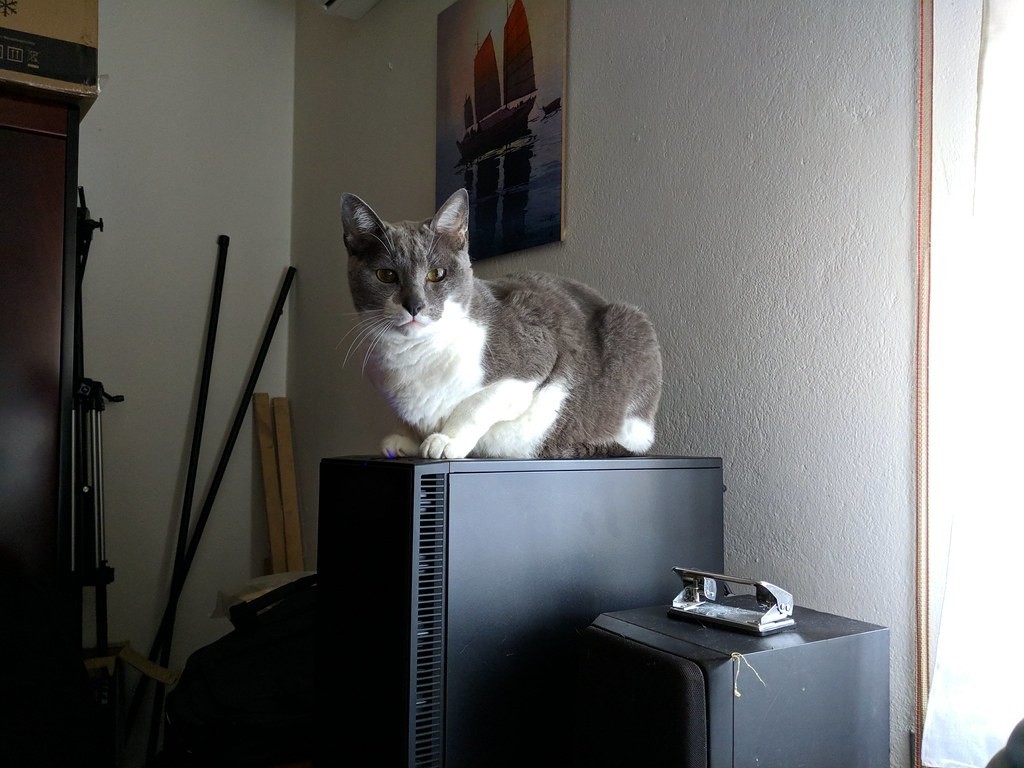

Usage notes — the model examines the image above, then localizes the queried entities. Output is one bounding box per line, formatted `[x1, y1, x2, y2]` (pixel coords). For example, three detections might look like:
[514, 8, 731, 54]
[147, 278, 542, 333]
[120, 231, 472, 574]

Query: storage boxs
[0, 0, 100, 122]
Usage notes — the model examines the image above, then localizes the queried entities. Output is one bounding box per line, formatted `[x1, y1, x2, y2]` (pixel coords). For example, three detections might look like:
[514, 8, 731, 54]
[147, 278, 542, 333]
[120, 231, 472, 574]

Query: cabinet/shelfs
[0, 81, 83, 768]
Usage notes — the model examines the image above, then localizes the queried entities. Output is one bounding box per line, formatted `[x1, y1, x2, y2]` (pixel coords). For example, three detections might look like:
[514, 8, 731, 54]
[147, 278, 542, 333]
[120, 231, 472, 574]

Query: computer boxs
[299, 453, 723, 768]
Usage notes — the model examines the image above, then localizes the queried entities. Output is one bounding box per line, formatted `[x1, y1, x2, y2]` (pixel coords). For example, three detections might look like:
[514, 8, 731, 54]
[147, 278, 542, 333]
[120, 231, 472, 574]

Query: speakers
[585, 600, 891, 768]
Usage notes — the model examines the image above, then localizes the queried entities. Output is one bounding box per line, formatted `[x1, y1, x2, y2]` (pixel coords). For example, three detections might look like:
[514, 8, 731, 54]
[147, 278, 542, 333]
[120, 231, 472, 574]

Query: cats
[335, 187, 663, 459]
